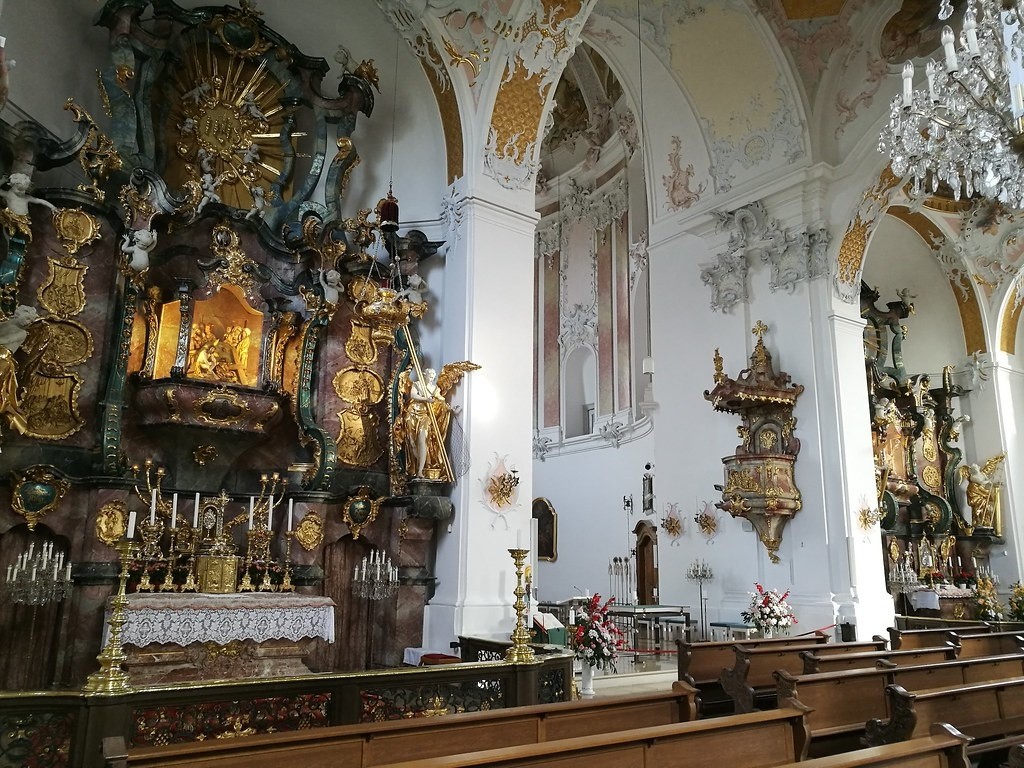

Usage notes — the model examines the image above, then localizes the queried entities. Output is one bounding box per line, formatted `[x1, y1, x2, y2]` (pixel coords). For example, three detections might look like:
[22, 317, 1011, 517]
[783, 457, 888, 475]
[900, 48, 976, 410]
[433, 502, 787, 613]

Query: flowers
[740, 582, 799, 633]
[954, 604, 966, 617]
[1006, 580, 1024, 621]
[925, 572, 945, 583]
[975, 578, 1004, 615]
[955, 572, 976, 584]
[570, 592, 625, 673]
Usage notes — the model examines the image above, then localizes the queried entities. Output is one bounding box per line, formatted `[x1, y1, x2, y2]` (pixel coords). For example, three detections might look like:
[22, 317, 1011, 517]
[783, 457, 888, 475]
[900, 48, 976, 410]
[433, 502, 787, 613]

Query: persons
[896, 288, 918, 315]
[875, 398, 890, 431]
[924, 409, 937, 431]
[178, 79, 270, 220]
[317, 266, 344, 322]
[395, 273, 429, 305]
[405, 369, 445, 478]
[966, 463, 995, 528]
[0, 304, 37, 435]
[186, 322, 251, 382]
[121, 230, 158, 292]
[0, 173, 59, 244]
[334, 45, 382, 95]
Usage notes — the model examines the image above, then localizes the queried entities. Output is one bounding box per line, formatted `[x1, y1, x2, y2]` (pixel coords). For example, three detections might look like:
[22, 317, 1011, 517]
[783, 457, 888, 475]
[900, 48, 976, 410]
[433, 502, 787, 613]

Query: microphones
[574, 586, 583, 597]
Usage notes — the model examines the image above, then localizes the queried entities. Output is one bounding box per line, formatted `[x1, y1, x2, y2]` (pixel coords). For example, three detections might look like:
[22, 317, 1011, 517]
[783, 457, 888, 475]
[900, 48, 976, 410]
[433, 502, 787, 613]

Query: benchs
[103, 618, 1024, 768]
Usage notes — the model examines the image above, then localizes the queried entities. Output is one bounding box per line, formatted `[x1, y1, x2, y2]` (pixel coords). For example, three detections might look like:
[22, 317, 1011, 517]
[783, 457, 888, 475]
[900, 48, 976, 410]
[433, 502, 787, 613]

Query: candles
[127, 511, 137, 538]
[354, 548, 398, 583]
[948, 557, 953, 568]
[171, 492, 177, 528]
[150, 487, 157, 525]
[971, 557, 1000, 583]
[888, 563, 918, 584]
[928, 555, 933, 567]
[192, 493, 200, 527]
[287, 498, 293, 533]
[6, 540, 73, 582]
[248, 495, 254, 529]
[516, 525, 523, 547]
[958, 556, 962, 567]
[688, 557, 713, 578]
[268, 495, 274, 530]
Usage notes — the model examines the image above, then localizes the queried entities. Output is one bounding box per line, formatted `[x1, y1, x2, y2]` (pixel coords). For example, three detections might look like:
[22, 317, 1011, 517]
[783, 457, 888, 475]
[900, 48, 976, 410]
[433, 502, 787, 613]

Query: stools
[549, 604, 565, 622]
[537, 603, 548, 615]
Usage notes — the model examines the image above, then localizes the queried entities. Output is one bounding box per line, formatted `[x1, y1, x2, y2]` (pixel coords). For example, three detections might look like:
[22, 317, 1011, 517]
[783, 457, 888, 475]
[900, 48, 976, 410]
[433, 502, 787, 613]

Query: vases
[578, 659, 595, 699]
[935, 583, 940, 588]
[959, 582, 966, 588]
[928, 584, 930, 587]
[969, 584, 977, 588]
[981, 610, 993, 620]
[764, 624, 773, 638]
[954, 616, 962, 620]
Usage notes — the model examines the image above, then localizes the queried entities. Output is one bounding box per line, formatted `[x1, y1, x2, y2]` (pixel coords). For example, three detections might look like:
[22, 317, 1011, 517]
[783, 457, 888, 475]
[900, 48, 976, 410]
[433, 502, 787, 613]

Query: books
[533, 613, 565, 632]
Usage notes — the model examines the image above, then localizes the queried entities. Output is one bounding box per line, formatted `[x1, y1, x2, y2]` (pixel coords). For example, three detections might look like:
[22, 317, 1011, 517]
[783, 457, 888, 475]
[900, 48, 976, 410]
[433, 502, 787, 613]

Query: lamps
[637, 1, 655, 375]
[878, 0, 1023, 210]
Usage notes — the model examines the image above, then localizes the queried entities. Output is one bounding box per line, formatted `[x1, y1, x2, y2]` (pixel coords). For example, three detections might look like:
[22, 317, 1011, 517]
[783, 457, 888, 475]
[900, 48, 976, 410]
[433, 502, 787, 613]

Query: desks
[102, 594, 335, 680]
[602, 604, 691, 663]
